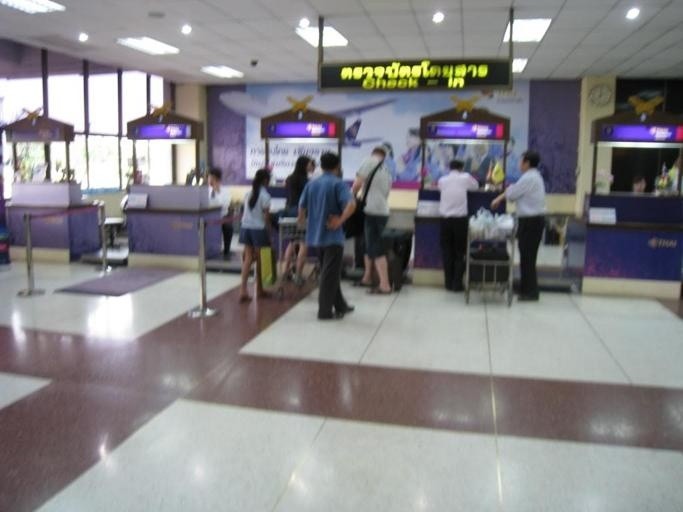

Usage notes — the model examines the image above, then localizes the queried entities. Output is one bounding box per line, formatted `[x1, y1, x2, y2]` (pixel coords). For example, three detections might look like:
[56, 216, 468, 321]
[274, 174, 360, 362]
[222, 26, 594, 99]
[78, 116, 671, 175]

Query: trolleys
[460, 207, 517, 308]
[275, 213, 325, 302]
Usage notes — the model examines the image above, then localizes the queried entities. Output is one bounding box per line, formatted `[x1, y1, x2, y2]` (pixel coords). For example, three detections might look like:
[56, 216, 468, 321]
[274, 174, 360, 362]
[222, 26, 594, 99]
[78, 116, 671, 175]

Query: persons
[629, 174, 646, 194]
[275, 145, 393, 321]
[238, 170, 274, 303]
[205, 166, 235, 259]
[490, 150, 547, 302]
[436, 157, 480, 293]
[383, 126, 523, 190]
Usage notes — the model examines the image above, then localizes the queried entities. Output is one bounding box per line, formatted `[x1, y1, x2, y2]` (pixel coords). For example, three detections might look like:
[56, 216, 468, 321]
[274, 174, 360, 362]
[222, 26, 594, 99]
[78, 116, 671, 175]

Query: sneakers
[281, 271, 304, 285]
[319, 307, 353, 318]
[239, 289, 271, 301]
[353, 280, 391, 294]
[514, 285, 539, 300]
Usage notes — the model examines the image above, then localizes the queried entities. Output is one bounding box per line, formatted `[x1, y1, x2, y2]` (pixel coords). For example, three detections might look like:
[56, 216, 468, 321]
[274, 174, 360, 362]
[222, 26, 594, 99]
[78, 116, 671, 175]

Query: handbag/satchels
[272, 211, 287, 228]
[342, 197, 366, 238]
[259, 245, 277, 287]
